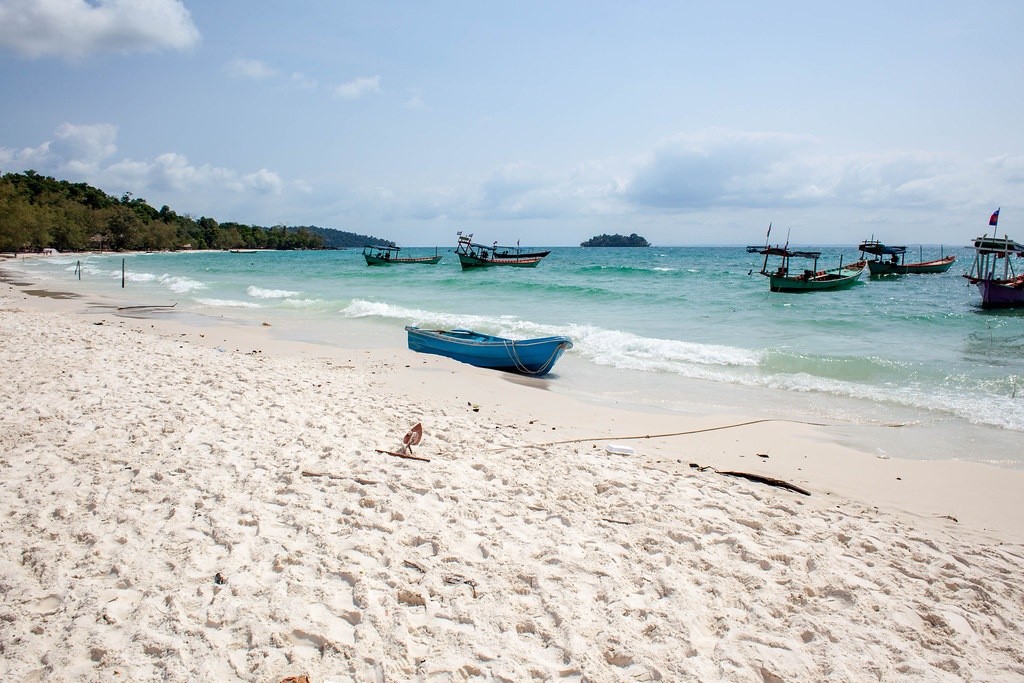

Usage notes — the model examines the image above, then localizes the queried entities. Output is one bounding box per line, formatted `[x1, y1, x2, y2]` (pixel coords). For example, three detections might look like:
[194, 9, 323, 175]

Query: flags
[989, 209, 999, 226]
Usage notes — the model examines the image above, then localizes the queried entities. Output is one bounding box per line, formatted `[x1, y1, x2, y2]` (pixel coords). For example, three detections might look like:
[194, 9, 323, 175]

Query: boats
[362, 243, 443, 267]
[404, 325, 574, 378]
[963, 234, 1024, 311]
[760, 221, 867, 293]
[455, 230, 552, 269]
[866, 239, 956, 276]
[858, 233, 909, 255]
[230, 249, 258, 253]
[746, 245, 821, 259]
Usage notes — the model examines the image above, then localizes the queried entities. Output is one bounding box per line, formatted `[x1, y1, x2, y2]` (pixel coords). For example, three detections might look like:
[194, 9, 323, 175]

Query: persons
[804, 270, 808, 277]
[15, 253, 16, 258]
[891, 254, 895, 262]
[43, 250, 52, 255]
[483, 251, 487, 258]
[385, 252, 390, 258]
